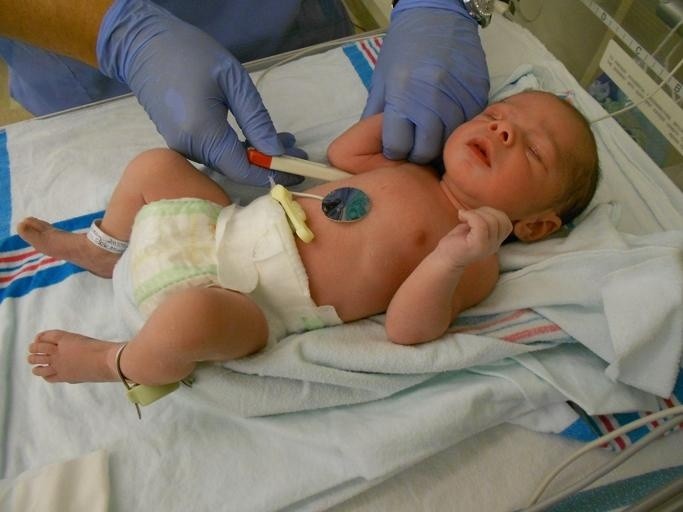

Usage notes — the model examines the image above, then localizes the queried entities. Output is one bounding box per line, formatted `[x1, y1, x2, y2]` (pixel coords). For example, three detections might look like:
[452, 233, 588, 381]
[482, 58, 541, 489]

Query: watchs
[461, 0, 492, 27]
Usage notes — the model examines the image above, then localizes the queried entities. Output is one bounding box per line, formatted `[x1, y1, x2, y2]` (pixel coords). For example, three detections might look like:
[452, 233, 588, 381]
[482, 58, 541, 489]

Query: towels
[112, 64, 683, 416]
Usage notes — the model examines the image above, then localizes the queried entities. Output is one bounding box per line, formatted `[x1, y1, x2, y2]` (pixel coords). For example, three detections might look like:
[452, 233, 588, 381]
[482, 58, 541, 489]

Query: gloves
[360, 0, 491, 165]
[96, 0, 309, 189]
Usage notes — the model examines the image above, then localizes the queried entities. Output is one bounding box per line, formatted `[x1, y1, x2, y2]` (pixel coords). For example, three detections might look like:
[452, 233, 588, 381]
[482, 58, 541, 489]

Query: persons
[14, 89, 602, 388]
[1, 0, 493, 187]
